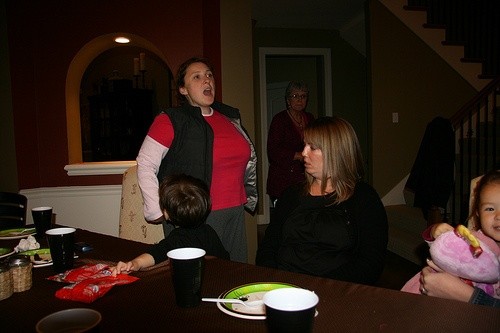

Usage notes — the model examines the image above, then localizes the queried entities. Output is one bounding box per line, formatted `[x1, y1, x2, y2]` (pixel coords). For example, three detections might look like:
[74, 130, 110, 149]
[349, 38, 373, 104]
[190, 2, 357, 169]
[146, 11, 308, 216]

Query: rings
[421, 288, 424, 293]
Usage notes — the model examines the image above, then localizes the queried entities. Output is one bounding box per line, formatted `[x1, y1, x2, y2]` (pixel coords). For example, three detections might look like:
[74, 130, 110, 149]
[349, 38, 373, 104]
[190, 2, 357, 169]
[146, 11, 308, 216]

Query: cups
[32, 206, 53, 231]
[35, 308, 102, 333]
[264, 287, 319, 333]
[167, 247, 206, 309]
[45, 228, 76, 272]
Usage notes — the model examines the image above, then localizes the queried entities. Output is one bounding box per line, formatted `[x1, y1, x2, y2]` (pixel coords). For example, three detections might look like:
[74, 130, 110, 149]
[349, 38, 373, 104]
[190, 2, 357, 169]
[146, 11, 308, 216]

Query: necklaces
[288, 109, 304, 127]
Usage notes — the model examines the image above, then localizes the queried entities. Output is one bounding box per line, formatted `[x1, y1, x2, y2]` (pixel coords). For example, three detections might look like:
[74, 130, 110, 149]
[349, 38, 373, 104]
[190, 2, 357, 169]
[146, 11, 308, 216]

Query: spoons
[202, 298, 262, 306]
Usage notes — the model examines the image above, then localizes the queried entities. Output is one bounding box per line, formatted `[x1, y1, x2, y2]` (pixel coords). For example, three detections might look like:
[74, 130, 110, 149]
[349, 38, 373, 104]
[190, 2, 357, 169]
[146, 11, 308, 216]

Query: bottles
[0, 259, 14, 300]
[8, 254, 33, 293]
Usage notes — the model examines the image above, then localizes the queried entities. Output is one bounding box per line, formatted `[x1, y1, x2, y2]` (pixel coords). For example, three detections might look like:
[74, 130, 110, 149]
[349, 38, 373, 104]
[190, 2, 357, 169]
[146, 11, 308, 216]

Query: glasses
[288, 93, 308, 99]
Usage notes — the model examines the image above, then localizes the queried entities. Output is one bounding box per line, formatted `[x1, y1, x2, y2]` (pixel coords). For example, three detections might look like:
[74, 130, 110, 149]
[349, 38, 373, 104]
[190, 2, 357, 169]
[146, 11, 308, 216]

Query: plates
[0, 247, 16, 258]
[0, 228, 36, 239]
[18, 248, 53, 267]
[216, 282, 303, 320]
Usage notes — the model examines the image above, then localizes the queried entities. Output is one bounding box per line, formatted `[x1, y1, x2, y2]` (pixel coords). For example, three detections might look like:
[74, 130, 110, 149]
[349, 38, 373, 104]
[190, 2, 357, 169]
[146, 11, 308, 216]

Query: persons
[264, 81, 317, 216]
[400, 170, 500, 308]
[136, 59, 259, 263]
[254, 116, 388, 287]
[418, 259, 500, 307]
[112, 184, 230, 279]
